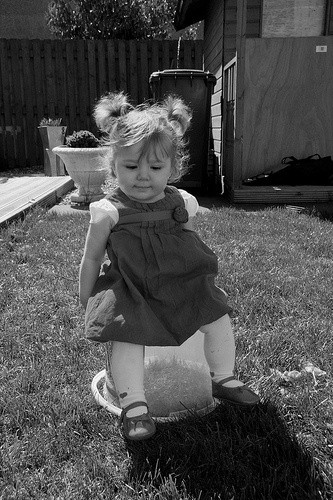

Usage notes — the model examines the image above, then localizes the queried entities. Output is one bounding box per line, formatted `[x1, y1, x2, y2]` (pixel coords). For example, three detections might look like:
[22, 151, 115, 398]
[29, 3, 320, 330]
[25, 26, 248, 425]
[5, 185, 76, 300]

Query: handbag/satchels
[282, 154, 333, 187]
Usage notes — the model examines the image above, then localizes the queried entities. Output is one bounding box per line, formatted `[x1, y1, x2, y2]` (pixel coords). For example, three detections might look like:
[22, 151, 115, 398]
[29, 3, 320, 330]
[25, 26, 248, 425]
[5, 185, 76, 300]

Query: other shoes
[121, 401, 155, 439]
[211, 376, 260, 405]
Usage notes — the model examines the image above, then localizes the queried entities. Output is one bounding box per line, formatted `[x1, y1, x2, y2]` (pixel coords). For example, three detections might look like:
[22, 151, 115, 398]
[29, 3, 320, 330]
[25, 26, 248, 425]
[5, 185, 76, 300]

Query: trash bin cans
[149, 68, 221, 198]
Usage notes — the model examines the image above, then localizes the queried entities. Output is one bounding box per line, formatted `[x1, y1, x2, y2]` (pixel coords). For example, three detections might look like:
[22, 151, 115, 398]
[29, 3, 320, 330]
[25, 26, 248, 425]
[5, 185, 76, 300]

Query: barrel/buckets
[91, 328, 229, 424]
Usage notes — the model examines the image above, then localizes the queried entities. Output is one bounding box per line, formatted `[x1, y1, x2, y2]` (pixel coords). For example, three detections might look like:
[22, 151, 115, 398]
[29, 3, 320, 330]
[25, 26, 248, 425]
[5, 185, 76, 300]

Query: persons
[79, 91, 261, 440]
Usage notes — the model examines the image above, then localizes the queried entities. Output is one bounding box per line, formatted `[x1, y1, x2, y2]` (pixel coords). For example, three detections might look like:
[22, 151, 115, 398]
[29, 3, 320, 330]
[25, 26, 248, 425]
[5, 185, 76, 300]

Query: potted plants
[51, 131, 112, 203]
[37, 118, 67, 177]
